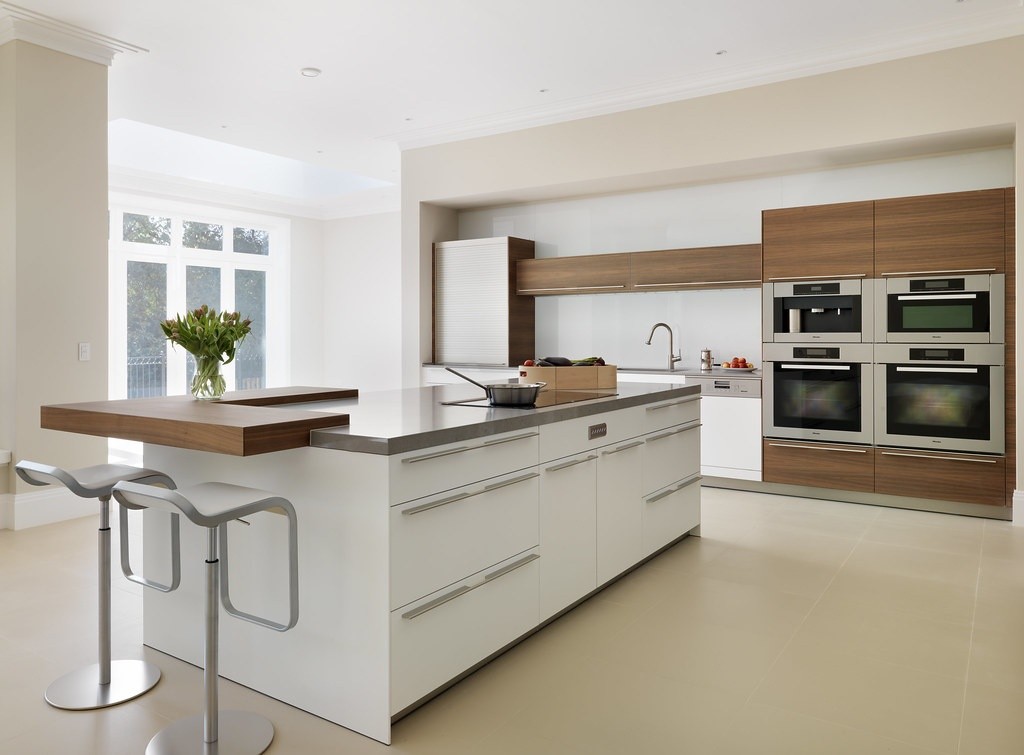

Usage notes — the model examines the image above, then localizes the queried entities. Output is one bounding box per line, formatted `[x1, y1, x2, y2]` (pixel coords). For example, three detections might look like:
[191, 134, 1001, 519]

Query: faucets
[646, 322, 682, 371]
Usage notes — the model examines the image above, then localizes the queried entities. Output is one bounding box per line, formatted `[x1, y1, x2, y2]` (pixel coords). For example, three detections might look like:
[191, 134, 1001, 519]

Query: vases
[190, 355, 225, 401]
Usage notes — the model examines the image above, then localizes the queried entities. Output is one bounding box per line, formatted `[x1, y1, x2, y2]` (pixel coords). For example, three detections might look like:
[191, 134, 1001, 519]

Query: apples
[722, 357, 752, 368]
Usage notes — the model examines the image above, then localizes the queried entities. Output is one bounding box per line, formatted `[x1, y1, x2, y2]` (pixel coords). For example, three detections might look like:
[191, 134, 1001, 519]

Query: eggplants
[533, 356, 592, 367]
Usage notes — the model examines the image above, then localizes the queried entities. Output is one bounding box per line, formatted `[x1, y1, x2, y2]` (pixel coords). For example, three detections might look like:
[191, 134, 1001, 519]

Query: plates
[722, 367, 758, 372]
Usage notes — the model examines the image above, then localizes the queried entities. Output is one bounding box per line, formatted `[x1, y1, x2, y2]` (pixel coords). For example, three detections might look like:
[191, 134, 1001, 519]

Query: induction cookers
[441, 389, 619, 410]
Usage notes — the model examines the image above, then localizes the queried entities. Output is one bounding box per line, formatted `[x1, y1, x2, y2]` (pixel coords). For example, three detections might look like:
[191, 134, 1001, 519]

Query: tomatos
[521, 360, 534, 377]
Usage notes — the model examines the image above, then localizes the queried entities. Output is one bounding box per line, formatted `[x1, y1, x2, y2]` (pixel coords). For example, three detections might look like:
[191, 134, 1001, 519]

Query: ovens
[762, 267, 1007, 454]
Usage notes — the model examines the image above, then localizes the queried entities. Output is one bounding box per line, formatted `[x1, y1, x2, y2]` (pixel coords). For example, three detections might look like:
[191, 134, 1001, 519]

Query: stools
[15, 458, 182, 712]
[112, 476, 298, 755]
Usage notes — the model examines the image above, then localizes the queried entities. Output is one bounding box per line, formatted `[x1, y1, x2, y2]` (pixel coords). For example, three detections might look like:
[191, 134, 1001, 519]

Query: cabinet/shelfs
[760, 187, 1004, 283]
[516, 243, 763, 293]
[535, 404, 646, 465]
[642, 421, 703, 562]
[310, 428, 540, 716]
[539, 434, 642, 629]
[431, 235, 535, 367]
[762, 437, 1006, 510]
[644, 389, 701, 435]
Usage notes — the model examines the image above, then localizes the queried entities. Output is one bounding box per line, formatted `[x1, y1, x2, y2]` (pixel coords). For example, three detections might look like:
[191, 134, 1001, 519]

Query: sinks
[617, 367, 667, 371]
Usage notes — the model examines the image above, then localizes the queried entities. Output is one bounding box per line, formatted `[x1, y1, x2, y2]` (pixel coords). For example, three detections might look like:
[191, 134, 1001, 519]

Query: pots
[446, 367, 547, 405]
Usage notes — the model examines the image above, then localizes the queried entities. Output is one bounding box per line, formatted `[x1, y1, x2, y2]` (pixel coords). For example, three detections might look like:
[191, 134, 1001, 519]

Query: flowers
[159, 304, 259, 397]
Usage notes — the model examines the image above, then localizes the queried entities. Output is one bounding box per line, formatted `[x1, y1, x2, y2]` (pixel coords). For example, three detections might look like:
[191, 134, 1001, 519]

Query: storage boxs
[518, 365, 617, 390]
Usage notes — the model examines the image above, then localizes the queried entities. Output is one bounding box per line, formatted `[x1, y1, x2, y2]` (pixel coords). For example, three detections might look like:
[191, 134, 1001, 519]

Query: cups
[700, 348, 714, 370]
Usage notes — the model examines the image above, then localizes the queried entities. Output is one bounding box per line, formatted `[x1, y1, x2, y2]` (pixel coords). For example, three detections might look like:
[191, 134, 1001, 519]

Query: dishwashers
[685, 370, 763, 481]
